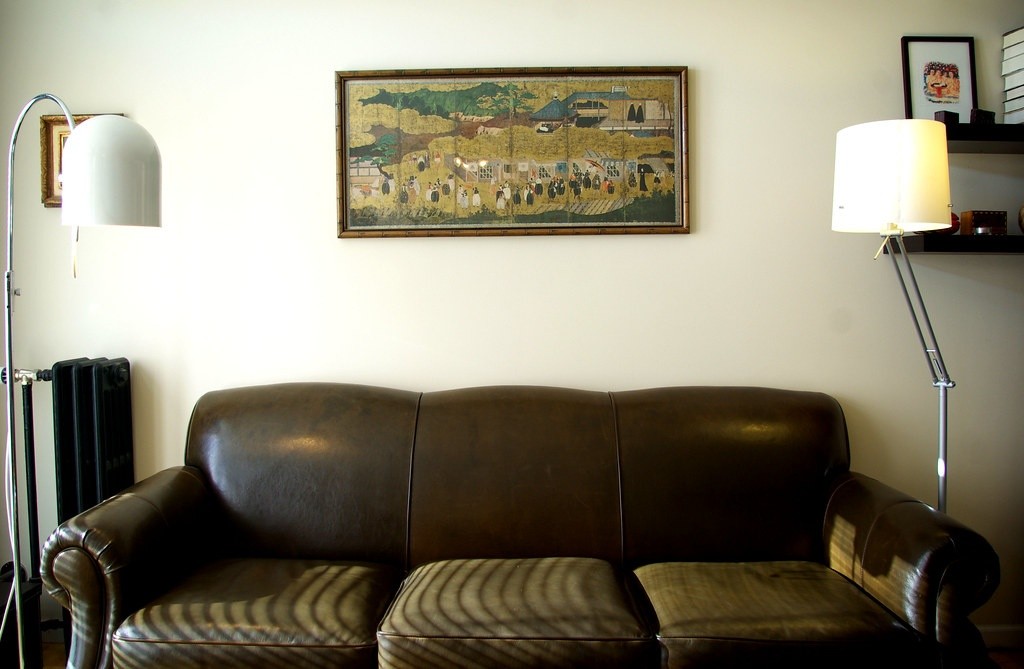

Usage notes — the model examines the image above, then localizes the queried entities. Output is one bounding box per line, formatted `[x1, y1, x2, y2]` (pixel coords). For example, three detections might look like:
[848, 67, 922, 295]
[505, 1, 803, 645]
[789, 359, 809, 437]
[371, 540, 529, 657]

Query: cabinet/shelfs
[883, 123, 1024, 255]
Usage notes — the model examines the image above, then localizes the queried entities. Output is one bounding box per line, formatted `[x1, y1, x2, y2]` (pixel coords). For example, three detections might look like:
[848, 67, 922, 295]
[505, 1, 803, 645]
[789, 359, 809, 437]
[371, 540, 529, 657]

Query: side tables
[0, 582, 42, 669]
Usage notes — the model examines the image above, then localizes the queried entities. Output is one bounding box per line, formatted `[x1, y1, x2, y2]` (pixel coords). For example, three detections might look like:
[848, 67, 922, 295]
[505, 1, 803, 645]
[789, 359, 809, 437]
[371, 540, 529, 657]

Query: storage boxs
[960, 210, 1008, 236]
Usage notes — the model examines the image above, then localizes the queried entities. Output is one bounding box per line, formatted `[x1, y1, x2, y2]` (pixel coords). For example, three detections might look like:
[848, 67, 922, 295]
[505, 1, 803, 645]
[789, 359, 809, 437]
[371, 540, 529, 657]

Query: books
[1001, 27, 1024, 124]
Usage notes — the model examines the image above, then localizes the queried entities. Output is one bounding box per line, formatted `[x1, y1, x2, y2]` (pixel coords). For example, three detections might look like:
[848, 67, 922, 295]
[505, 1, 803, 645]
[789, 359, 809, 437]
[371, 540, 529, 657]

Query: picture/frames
[39, 112, 124, 208]
[334, 65, 691, 238]
[901, 35, 979, 123]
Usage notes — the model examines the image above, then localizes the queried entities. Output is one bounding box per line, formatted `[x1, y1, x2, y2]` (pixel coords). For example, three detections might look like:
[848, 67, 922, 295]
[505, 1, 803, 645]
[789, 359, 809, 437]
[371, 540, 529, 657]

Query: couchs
[39, 382, 1001, 669]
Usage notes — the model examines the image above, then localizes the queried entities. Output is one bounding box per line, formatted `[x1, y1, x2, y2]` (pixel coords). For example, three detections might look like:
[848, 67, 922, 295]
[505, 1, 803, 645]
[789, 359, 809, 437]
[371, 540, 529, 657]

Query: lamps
[4, 93, 163, 669]
[831, 118, 957, 511]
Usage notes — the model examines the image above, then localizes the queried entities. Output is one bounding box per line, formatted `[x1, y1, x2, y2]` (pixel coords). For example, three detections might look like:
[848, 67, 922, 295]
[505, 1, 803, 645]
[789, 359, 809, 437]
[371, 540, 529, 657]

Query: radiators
[0, 357, 135, 669]
